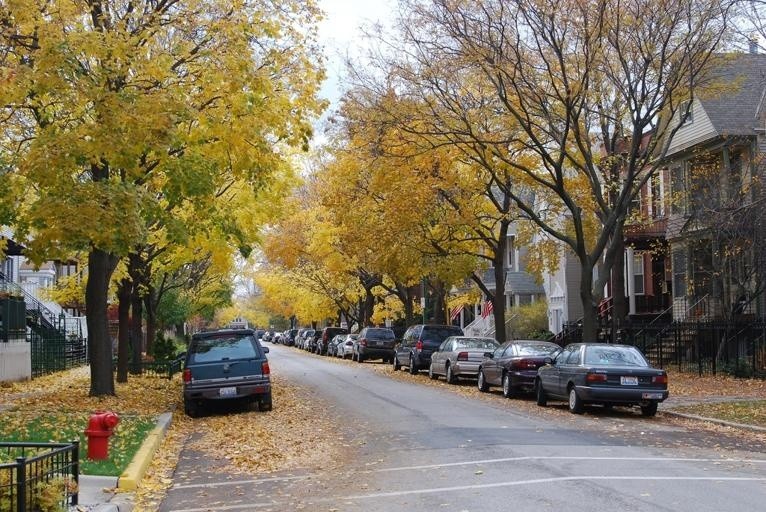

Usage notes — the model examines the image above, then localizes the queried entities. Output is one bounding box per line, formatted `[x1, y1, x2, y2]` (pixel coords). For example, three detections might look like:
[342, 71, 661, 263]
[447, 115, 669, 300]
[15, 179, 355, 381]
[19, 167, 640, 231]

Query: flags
[450, 303, 464, 320]
[481, 298, 494, 319]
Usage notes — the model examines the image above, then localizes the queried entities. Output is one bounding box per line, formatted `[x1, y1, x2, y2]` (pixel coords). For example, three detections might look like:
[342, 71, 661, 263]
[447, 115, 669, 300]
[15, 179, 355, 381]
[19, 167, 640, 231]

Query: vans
[182, 327, 272, 417]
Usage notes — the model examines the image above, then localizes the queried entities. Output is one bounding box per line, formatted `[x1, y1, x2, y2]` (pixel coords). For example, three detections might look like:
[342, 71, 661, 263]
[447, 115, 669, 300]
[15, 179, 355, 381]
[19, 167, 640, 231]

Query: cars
[352, 326, 397, 365]
[477, 339, 563, 399]
[255, 327, 359, 358]
[429, 335, 501, 385]
[392, 324, 465, 375]
[535, 341, 669, 416]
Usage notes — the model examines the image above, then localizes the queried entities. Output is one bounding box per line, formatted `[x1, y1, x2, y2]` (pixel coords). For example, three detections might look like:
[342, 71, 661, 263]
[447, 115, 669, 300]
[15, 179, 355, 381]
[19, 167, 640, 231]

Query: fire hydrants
[84, 410, 120, 460]
